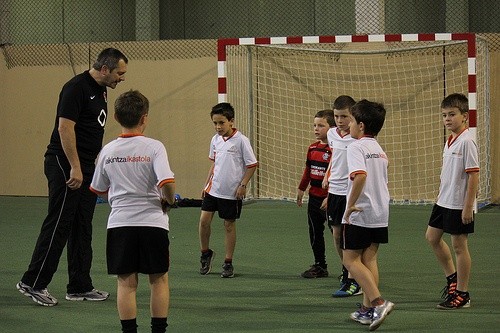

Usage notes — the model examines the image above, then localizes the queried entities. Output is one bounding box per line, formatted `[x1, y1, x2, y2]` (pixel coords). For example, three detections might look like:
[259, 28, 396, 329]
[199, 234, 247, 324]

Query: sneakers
[16, 280, 58, 307]
[65, 288, 110, 300]
[301, 263, 328, 278]
[220, 261, 235, 278]
[436, 289, 473, 309]
[199, 249, 216, 275]
[332, 281, 363, 297]
[365, 299, 394, 330]
[440, 280, 457, 303]
[349, 303, 372, 324]
[338, 267, 348, 287]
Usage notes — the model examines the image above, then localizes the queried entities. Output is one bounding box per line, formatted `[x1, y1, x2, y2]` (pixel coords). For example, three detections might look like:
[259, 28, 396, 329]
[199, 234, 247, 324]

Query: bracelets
[240, 183, 246, 189]
[166, 202, 174, 208]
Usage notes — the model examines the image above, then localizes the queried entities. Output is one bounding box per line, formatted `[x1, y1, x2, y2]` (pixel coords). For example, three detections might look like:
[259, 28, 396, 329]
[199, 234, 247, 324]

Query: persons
[16, 48, 129, 306]
[425, 93, 480, 309]
[340, 99, 395, 332]
[322, 95, 363, 298]
[198, 103, 258, 278]
[89, 89, 175, 333]
[297, 110, 337, 278]
